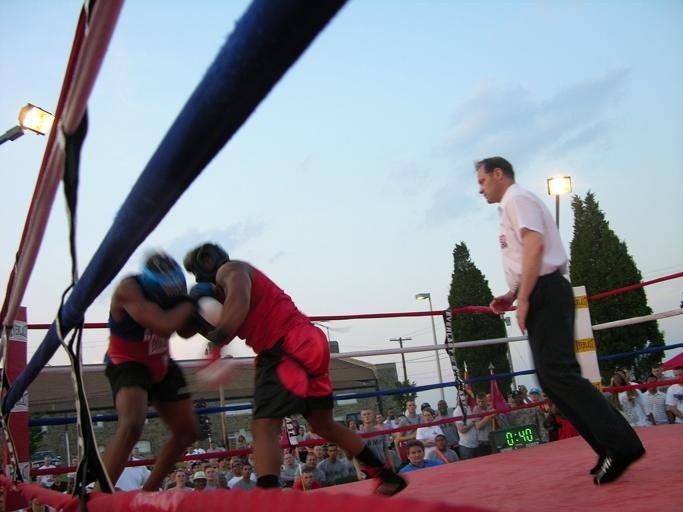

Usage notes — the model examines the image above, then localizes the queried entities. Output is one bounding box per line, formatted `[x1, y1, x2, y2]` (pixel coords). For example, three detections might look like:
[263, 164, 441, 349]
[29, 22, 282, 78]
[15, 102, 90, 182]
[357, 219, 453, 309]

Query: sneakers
[592, 440, 649, 486]
[587, 452, 605, 474]
[371, 470, 410, 499]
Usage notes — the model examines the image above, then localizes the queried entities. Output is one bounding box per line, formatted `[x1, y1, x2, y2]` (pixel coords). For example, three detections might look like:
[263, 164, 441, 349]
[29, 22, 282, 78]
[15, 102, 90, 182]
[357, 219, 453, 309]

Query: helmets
[141, 253, 186, 306]
[183, 241, 229, 280]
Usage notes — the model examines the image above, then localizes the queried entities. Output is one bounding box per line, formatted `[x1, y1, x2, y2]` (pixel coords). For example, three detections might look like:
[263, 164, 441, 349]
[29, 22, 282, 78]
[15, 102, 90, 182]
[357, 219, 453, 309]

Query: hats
[420, 402, 431, 410]
[192, 471, 207, 481]
[529, 388, 540, 395]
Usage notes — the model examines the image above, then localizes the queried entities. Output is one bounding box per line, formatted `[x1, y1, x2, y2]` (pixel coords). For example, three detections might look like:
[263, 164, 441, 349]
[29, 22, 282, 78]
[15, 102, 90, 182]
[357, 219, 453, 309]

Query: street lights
[413, 291, 445, 403]
[547, 173, 571, 229]
[388, 337, 412, 396]
[0, 101, 54, 145]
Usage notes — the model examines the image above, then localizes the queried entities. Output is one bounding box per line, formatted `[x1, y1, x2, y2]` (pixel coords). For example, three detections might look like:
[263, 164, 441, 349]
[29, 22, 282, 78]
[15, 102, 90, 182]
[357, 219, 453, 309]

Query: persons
[93, 253, 218, 492]
[37, 387, 564, 497]
[476, 157, 647, 485]
[184, 242, 407, 497]
[609, 356, 683, 426]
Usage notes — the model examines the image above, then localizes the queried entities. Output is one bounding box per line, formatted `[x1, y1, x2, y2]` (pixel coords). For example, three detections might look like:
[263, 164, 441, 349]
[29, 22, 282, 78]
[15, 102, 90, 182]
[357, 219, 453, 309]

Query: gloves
[188, 282, 226, 305]
[193, 296, 223, 336]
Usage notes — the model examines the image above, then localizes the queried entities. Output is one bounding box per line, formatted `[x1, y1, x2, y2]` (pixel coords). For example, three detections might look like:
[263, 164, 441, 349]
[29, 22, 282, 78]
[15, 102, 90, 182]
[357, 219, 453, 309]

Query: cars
[29, 448, 63, 469]
